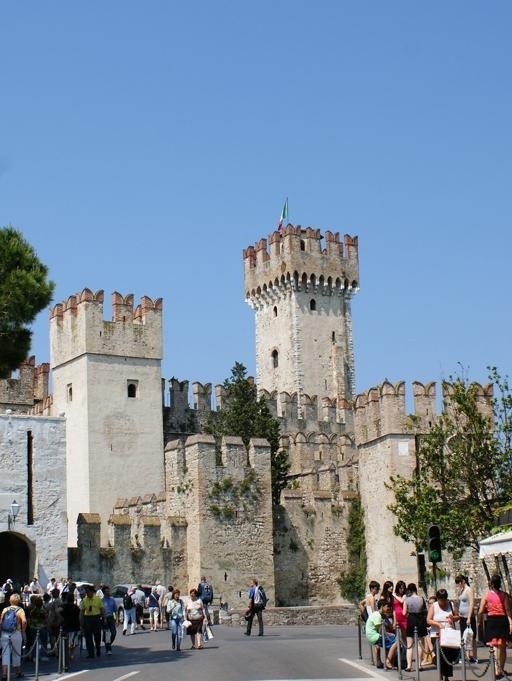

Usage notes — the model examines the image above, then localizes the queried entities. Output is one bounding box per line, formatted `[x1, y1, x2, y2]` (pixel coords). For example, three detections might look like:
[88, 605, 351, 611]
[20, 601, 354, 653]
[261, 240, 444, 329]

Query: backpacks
[0, 605, 22, 634]
[254, 585, 269, 610]
[5, 584, 11, 591]
[123, 592, 135, 610]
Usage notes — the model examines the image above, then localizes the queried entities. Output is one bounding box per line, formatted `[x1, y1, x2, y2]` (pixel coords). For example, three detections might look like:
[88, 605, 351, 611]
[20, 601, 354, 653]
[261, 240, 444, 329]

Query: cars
[47, 581, 104, 606]
[111, 582, 171, 625]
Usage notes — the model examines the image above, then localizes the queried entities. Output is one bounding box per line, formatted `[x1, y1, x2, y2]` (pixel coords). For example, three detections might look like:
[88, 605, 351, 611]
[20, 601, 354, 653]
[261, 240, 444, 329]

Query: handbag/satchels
[202, 620, 215, 644]
[420, 596, 432, 628]
[439, 617, 462, 649]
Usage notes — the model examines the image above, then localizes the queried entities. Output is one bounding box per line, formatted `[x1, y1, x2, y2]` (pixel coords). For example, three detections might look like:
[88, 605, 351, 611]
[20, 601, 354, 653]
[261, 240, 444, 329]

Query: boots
[1, 665, 8, 680]
[14, 665, 24, 678]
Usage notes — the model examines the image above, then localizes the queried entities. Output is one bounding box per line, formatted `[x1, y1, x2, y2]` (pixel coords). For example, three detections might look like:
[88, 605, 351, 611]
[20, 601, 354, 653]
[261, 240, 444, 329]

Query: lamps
[8, 499, 21, 524]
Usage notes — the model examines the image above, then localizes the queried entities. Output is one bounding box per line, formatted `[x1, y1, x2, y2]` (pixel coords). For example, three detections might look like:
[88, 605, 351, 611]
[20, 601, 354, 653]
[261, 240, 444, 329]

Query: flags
[278, 200, 287, 230]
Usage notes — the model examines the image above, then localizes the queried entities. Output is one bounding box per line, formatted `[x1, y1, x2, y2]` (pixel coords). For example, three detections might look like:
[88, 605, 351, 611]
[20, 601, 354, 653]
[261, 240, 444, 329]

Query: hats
[6, 578, 13, 583]
[245, 611, 253, 622]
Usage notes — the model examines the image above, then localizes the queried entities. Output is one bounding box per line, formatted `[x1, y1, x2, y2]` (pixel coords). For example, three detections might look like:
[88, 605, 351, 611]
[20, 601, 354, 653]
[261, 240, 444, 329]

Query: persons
[244, 579, 263, 636]
[455, 575, 476, 663]
[323, 249, 327, 255]
[477, 575, 512, 679]
[359, 574, 461, 681]
[0, 576, 214, 681]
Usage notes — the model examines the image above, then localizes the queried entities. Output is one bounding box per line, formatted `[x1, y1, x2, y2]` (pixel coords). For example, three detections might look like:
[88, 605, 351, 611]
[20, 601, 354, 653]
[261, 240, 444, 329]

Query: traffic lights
[427, 524, 442, 562]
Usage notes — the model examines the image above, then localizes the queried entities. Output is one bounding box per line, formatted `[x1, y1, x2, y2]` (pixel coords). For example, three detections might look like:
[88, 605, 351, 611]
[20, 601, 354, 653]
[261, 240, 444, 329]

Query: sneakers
[191, 644, 204, 650]
[172, 643, 181, 652]
[244, 632, 251, 636]
[122, 624, 171, 636]
[496, 670, 509, 680]
[32, 651, 113, 664]
[258, 634, 264, 637]
[377, 663, 423, 672]
[465, 656, 481, 664]
